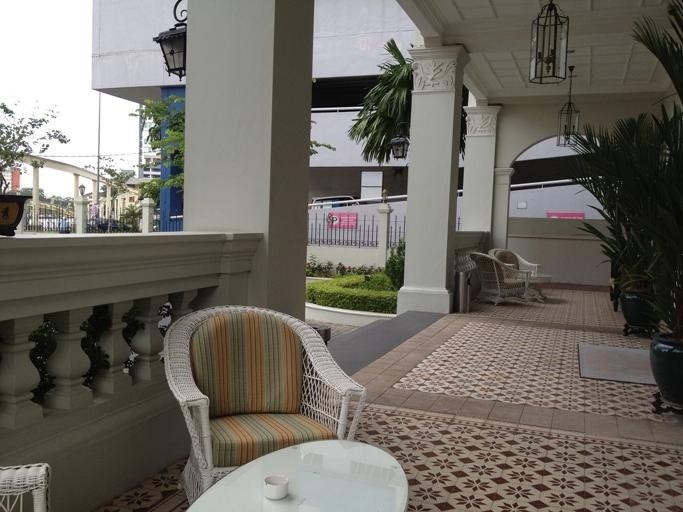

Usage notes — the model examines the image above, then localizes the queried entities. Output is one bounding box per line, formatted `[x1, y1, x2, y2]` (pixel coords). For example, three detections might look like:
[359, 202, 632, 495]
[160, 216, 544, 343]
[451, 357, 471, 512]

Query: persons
[58, 217, 72, 233]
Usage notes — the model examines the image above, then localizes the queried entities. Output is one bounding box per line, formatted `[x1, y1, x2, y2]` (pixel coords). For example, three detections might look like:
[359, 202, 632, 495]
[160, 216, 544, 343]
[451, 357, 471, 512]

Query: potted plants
[561, 101, 682, 405]
[0, 101, 69, 235]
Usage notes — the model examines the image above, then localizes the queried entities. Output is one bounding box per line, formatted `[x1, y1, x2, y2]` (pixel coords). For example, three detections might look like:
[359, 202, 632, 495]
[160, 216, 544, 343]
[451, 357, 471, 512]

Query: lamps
[154, 0, 187, 82]
[388, 122, 410, 158]
[531, 1, 581, 147]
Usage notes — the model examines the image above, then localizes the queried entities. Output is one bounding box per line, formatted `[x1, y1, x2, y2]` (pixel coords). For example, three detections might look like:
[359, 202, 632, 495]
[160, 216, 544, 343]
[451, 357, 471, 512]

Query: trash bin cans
[300, 322, 331, 416]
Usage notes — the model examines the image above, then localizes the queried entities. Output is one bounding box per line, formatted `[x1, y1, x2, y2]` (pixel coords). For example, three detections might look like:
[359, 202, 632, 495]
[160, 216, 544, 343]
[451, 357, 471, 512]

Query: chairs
[163, 304, 368, 508]
[470, 248, 545, 307]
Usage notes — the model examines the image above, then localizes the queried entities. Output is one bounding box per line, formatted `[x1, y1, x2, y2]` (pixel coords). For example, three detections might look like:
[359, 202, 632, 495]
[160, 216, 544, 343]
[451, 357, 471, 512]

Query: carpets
[578, 342, 657, 386]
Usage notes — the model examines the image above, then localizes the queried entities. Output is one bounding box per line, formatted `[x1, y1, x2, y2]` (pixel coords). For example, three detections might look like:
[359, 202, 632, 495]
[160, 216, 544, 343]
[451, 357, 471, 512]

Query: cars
[152, 219, 159, 232]
[58, 217, 96, 233]
[99, 218, 130, 232]
